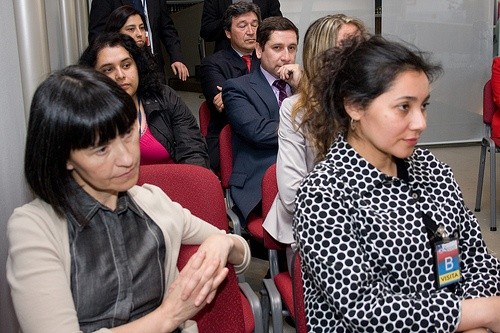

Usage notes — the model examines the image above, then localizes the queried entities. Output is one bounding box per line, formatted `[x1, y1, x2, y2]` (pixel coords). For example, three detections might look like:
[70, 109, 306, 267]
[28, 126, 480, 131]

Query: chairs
[475, 79, 500, 230]
[199, 101, 305, 333]
[136, 164, 263, 333]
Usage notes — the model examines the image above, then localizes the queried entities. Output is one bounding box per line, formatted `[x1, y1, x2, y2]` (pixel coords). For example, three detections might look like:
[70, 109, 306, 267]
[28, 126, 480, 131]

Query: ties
[242, 55, 252, 72]
[272, 80, 288, 106]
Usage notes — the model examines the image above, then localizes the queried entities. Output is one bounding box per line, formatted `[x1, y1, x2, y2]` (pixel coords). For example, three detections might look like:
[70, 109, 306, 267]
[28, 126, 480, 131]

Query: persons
[222, 16, 304, 224]
[77, 34, 208, 169]
[294, 34, 500, 333]
[88, 0, 189, 84]
[262, 16, 365, 246]
[491, 57, 500, 148]
[6, 65, 252, 332]
[200, 0, 283, 134]
